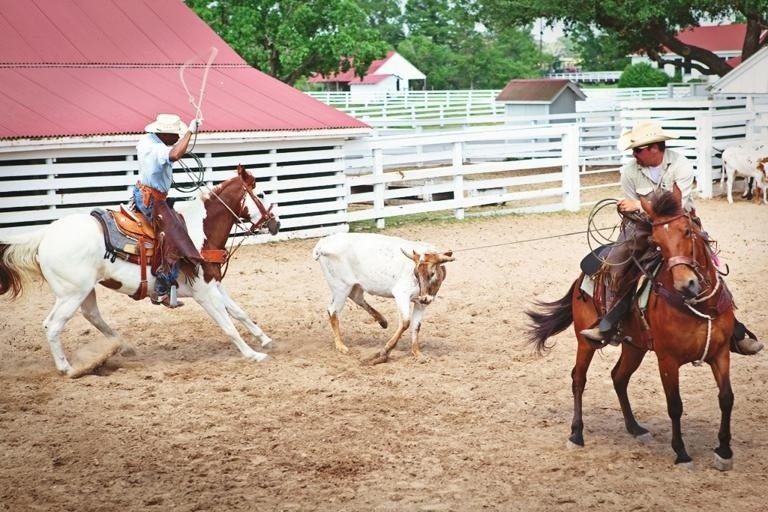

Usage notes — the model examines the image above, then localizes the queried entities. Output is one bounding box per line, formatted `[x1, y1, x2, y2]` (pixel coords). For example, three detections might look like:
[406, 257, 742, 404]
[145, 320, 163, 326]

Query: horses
[523, 180, 740, 472]
[0, 162, 283, 377]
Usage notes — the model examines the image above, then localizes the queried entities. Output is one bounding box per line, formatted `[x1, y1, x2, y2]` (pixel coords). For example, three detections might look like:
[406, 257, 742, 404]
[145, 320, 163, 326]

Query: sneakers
[580, 326, 623, 347]
[730, 337, 764, 354]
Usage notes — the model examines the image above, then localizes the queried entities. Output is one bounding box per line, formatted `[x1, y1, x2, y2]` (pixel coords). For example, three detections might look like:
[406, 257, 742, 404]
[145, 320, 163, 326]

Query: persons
[131, 112, 207, 309]
[579, 121, 764, 357]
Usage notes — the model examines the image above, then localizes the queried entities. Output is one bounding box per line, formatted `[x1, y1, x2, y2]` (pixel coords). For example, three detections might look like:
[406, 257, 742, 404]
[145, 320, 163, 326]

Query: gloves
[188, 119, 204, 134]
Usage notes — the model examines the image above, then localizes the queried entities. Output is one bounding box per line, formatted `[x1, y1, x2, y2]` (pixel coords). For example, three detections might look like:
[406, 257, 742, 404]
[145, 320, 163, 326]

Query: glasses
[633, 144, 654, 153]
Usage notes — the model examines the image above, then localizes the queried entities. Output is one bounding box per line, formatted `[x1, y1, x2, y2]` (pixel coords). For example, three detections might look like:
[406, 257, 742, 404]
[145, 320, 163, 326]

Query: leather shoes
[150, 291, 185, 308]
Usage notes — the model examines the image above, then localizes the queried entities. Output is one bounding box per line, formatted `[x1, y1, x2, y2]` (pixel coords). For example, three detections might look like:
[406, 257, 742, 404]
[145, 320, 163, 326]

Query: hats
[617, 120, 681, 152]
[143, 113, 189, 136]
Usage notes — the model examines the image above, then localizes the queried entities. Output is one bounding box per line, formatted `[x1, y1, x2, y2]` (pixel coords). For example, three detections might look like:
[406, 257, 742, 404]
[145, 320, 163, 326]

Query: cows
[310, 229, 457, 364]
[718, 146, 768, 206]
